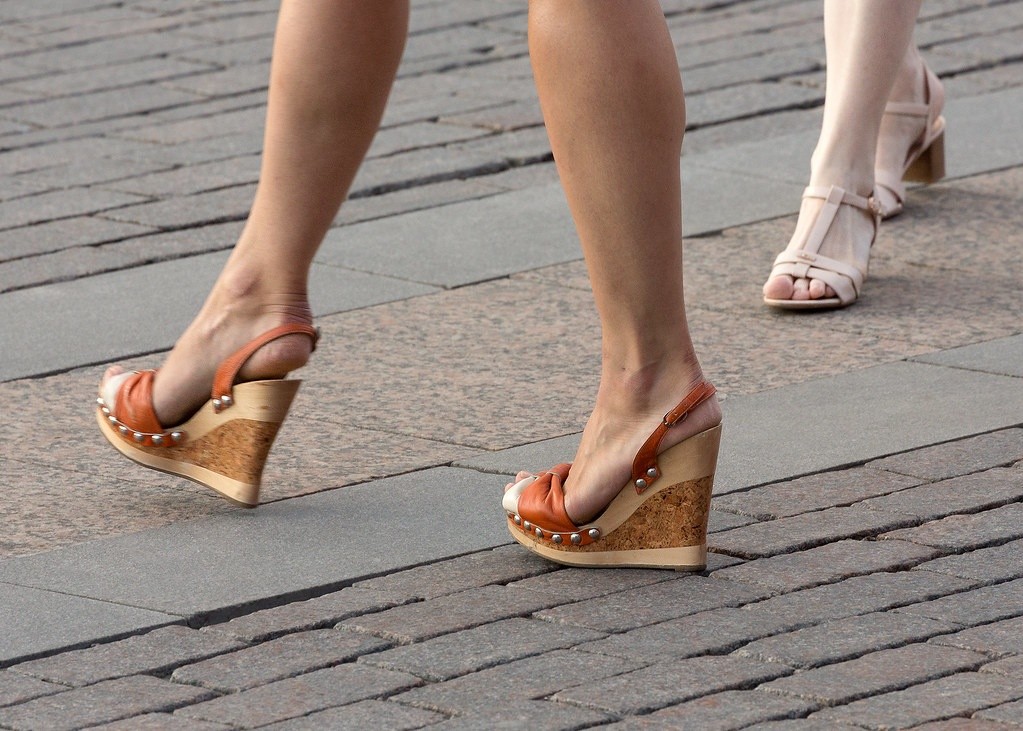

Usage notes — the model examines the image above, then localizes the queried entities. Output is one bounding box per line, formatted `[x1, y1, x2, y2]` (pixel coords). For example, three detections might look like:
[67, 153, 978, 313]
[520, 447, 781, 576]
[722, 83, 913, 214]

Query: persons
[95, 0, 722, 572]
[763, 0, 945, 307]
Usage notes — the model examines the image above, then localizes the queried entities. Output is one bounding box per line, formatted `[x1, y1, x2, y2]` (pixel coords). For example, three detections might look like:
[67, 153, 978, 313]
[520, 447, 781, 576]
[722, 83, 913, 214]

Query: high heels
[502, 381, 723, 577]
[96, 324, 322, 509]
[762, 185, 888, 310]
[872, 57, 947, 220]
